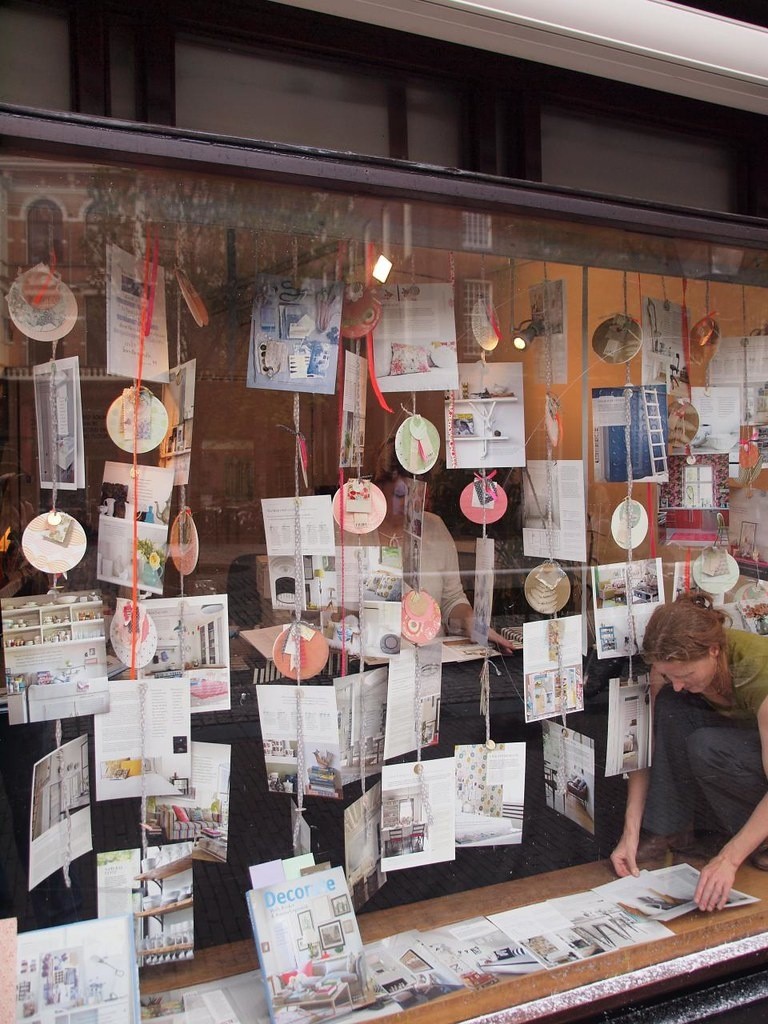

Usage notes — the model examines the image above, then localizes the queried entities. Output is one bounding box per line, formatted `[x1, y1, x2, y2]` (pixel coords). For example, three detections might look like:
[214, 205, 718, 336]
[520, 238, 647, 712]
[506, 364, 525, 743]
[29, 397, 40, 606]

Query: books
[244, 865, 376, 1024]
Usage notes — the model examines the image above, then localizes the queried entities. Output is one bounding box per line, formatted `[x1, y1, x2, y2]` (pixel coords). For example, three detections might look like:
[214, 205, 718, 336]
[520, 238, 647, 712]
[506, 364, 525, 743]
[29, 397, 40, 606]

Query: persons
[325, 437, 515, 648]
[606, 591, 767, 912]
[11, 499, 130, 750]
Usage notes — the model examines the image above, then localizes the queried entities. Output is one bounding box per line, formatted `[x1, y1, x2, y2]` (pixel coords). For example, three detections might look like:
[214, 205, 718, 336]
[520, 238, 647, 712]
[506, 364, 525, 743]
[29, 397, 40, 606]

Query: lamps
[513, 320, 545, 350]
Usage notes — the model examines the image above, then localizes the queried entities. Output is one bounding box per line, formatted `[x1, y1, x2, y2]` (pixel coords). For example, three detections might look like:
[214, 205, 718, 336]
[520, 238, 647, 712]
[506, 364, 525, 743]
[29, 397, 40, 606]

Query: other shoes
[749, 842, 768, 871]
[666, 827, 728, 857]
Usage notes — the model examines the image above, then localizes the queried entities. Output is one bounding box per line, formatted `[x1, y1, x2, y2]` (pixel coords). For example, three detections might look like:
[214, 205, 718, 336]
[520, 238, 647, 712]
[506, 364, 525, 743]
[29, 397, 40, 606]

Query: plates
[459, 480, 509, 525]
[170, 512, 199, 575]
[106, 389, 169, 456]
[271, 626, 331, 680]
[337, 287, 381, 337]
[22, 511, 87, 572]
[591, 315, 644, 364]
[524, 563, 571, 616]
[332, 480, 387, 534]
[6, 272, 78, 341]
[396, 414, 441, 476]
[667, 400, 699, 448]
[472, 299, 502, 354]
[610, 499, 649, 551]
[688, 318, 721, 366]
[692, 550, 741, 594]
[400, 591, 442, 644]
[109, 604, 159, 669]
